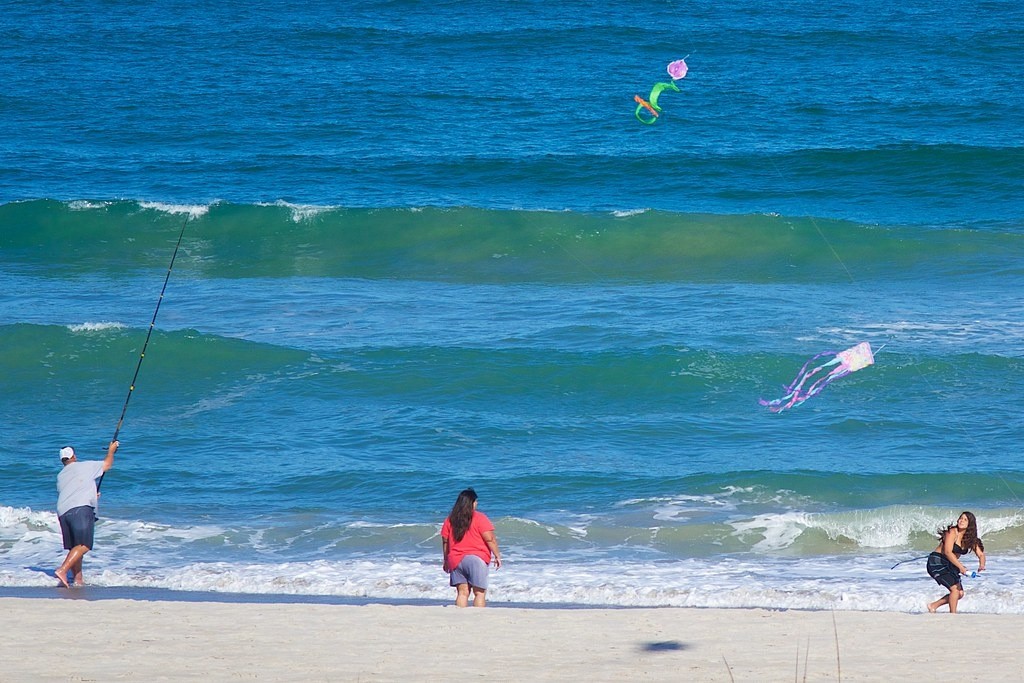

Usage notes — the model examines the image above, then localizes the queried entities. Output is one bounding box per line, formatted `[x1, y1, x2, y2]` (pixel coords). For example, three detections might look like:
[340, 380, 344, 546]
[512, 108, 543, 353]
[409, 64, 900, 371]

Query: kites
[759, 342, 874, 414]
[634, 60, 687, 125]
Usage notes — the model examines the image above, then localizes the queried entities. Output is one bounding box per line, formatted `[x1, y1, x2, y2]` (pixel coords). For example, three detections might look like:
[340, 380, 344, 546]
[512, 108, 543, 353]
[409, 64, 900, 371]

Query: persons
[441, 488, 501, 608]
[927, 511, 986, 613]
[54, 441, 118, 588]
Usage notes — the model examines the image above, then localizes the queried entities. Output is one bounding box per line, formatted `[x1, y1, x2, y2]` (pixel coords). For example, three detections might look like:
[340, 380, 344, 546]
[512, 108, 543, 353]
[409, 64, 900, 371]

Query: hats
[59, 446, 75, 461]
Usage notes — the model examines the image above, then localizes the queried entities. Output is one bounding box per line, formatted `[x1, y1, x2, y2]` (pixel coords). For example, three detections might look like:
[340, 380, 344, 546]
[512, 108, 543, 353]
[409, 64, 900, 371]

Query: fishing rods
[97, 214, 191, 495]
